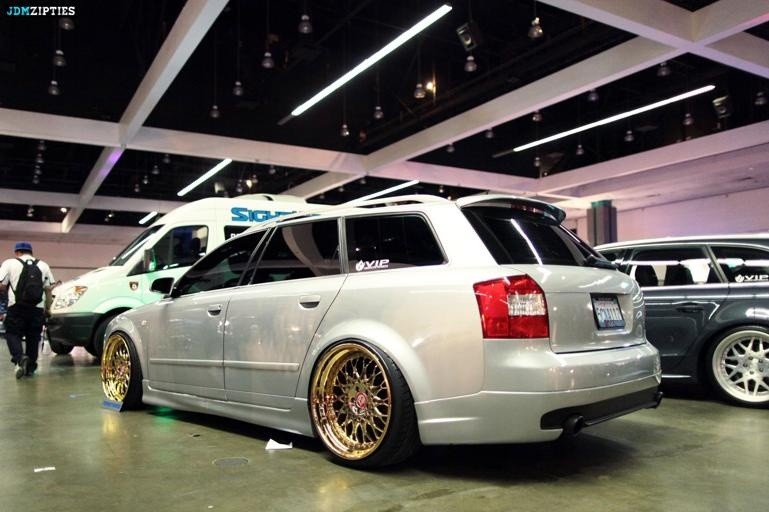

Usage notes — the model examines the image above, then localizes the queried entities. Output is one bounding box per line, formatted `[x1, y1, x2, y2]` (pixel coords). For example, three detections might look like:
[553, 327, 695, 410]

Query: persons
[0, 242, 55, 379]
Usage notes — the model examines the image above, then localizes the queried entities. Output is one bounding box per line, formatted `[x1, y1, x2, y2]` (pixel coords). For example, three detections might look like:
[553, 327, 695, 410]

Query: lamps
[24, 1, 768, 226]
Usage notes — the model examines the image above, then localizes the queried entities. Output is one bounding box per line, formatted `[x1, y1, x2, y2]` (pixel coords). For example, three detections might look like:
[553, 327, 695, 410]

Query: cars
[101, 178, 664, 471]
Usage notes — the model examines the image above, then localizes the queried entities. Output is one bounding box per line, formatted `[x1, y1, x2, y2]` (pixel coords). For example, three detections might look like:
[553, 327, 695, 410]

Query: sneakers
[14, 357, 33, 379]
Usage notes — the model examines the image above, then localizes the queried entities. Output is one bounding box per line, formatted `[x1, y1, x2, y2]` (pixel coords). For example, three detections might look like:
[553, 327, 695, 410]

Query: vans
[39, 193, 336, 364]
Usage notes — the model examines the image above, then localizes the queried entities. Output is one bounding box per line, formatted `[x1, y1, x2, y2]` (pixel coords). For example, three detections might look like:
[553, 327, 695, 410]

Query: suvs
[593, 233, 769, 409]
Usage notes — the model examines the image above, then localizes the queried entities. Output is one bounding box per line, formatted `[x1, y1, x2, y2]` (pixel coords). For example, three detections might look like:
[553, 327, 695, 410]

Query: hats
[15, 242, 32, 251]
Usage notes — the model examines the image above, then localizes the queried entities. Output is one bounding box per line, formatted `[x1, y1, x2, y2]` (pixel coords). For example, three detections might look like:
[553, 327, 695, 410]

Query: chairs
[663, 264, 692, 284]
[707, 262, 730, 283]
[221, 252, 274, 287]
[182, 237, 201, 267]
[634, 262, 658, 286]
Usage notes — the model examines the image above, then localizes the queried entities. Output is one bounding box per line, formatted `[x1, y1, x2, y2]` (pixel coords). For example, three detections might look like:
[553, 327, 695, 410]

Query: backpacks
[10, 257, 45, 307]
